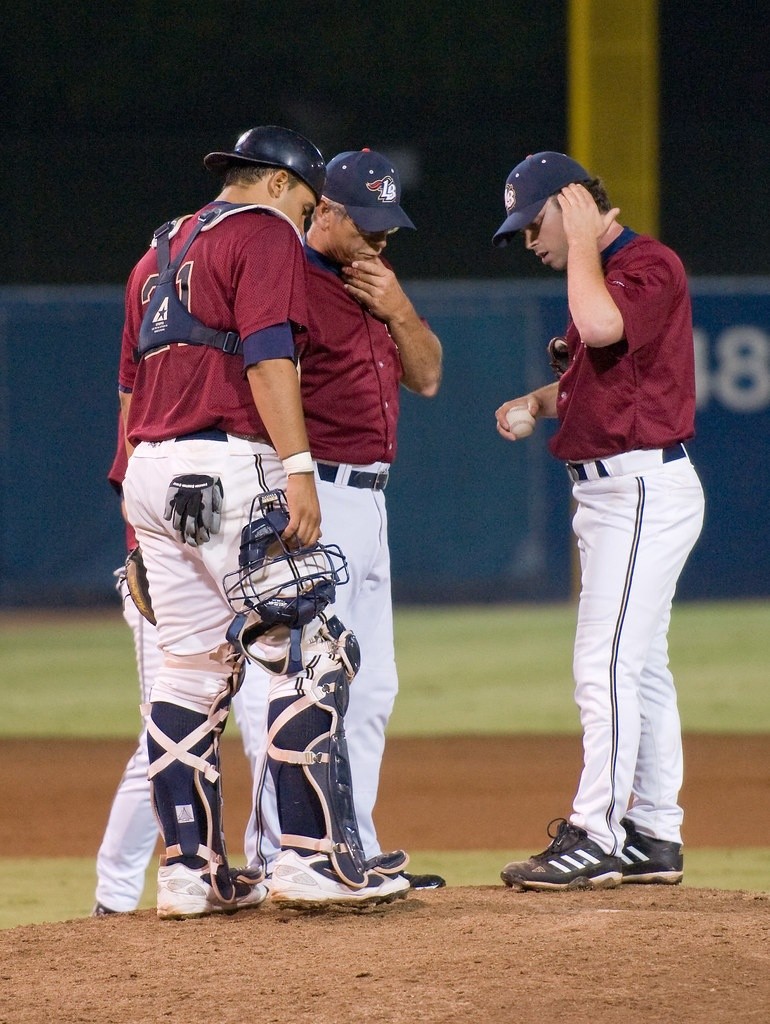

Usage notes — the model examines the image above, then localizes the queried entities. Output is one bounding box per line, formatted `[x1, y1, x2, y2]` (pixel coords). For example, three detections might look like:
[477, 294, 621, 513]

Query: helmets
[205, 125, 324, 204]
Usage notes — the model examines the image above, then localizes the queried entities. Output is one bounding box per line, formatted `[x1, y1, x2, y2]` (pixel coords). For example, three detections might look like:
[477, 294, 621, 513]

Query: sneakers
[156, 854, 269, 919]
[620, 818, 684, 884]
[270, 849, 410, 904]
[501, 818, 623, 889]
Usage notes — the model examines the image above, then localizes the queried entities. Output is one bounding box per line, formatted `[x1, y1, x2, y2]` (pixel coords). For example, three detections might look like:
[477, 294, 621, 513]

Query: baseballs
[506, 406, 535, 437]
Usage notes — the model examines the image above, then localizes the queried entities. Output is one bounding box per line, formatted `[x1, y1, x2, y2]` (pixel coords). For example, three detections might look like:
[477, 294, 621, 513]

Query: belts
[173, 426, 229, 442]
[566, 443, 688, 482]
[317, 463, 389, 491]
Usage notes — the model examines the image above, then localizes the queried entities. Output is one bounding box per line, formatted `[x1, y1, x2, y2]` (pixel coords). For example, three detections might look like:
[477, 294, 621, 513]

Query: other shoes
[95, 903, 111, 915]
[259, 877, 269, 886]
[404, 873, 445, 889]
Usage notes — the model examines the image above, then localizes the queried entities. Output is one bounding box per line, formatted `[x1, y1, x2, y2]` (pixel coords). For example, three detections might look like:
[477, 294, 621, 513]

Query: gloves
[164, 475, 224, 547]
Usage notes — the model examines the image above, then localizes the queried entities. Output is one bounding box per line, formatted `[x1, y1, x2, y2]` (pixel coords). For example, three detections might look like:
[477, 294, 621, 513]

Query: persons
[494, 150, 706, 891]
[94, 125, 446, 923]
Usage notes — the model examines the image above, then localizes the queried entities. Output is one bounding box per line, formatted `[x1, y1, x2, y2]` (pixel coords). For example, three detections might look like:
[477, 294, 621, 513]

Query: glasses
[330, 204, 402, 234]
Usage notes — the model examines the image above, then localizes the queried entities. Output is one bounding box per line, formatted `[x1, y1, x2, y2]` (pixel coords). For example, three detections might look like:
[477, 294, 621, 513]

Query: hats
[323, 147, 418, 230]
[492, 152, 587, 249]
